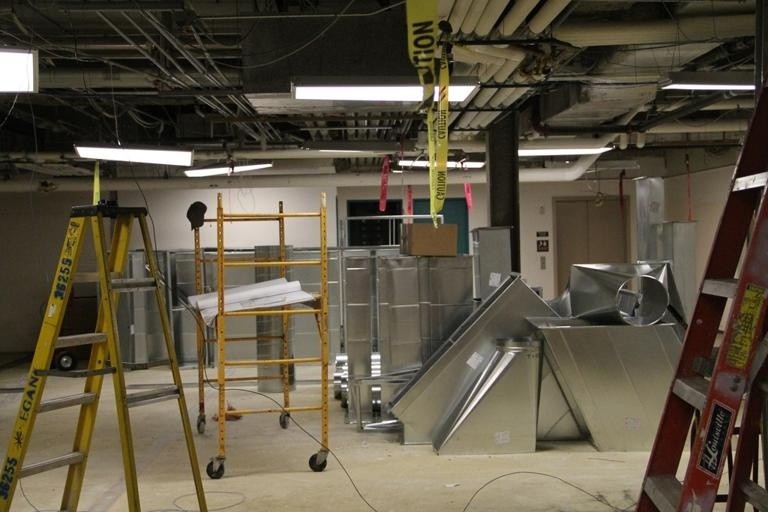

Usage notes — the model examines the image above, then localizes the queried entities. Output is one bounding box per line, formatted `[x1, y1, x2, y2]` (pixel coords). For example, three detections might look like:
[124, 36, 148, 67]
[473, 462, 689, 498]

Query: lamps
[73, 64, 193, 166]
[396, 156, 486, 170]
[0, 46, 39, 93]
[291, 74, 480, 103]
[183, 140, 274, 178]
[657, 0, 755, 91]
[518, 142, 615, 157]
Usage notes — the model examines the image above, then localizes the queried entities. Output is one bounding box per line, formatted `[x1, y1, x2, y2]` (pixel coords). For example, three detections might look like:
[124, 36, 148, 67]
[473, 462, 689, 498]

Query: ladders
[0, 189, 208, 512]
[631, 75, 768, 512]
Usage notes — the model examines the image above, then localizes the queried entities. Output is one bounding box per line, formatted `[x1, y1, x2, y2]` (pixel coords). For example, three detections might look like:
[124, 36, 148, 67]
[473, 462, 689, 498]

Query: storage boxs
[405, 223, 458, 258]
[400, 223, 406, 253]
